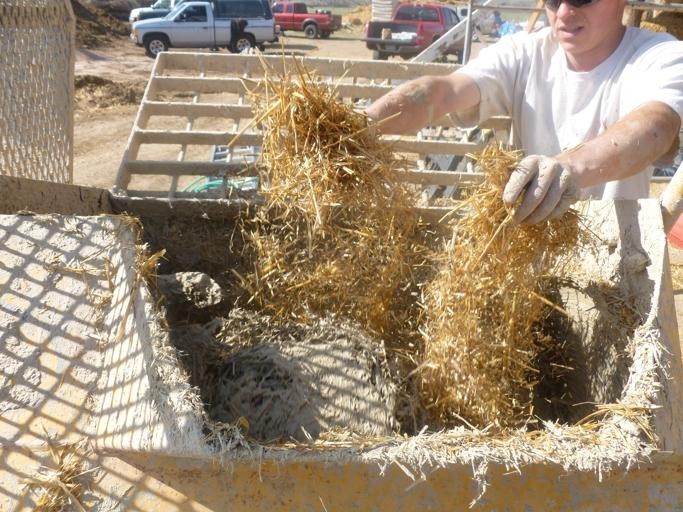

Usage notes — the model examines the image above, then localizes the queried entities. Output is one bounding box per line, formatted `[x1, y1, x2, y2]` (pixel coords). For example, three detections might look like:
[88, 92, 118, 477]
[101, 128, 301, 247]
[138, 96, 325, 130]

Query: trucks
[451, 6, 497, 42]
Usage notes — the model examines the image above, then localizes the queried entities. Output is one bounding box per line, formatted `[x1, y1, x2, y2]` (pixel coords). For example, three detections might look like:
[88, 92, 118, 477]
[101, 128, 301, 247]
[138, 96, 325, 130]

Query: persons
[349, 0, 683, 229]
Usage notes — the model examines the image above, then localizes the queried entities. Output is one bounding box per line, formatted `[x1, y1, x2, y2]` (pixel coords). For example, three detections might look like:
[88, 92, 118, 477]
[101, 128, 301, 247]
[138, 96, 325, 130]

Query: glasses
[543, 0, 598, 9]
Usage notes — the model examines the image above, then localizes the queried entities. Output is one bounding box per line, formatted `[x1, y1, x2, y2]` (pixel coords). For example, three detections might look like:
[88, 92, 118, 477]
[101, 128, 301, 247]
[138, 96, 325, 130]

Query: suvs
[128, 1, 184, 30]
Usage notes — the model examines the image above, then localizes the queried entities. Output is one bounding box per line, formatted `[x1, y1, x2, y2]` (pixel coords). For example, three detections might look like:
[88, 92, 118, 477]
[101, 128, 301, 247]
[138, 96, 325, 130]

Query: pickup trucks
[271, 2, 342, 39]
[361, 3, 465, 64]
[131, 0, 280, 58]
